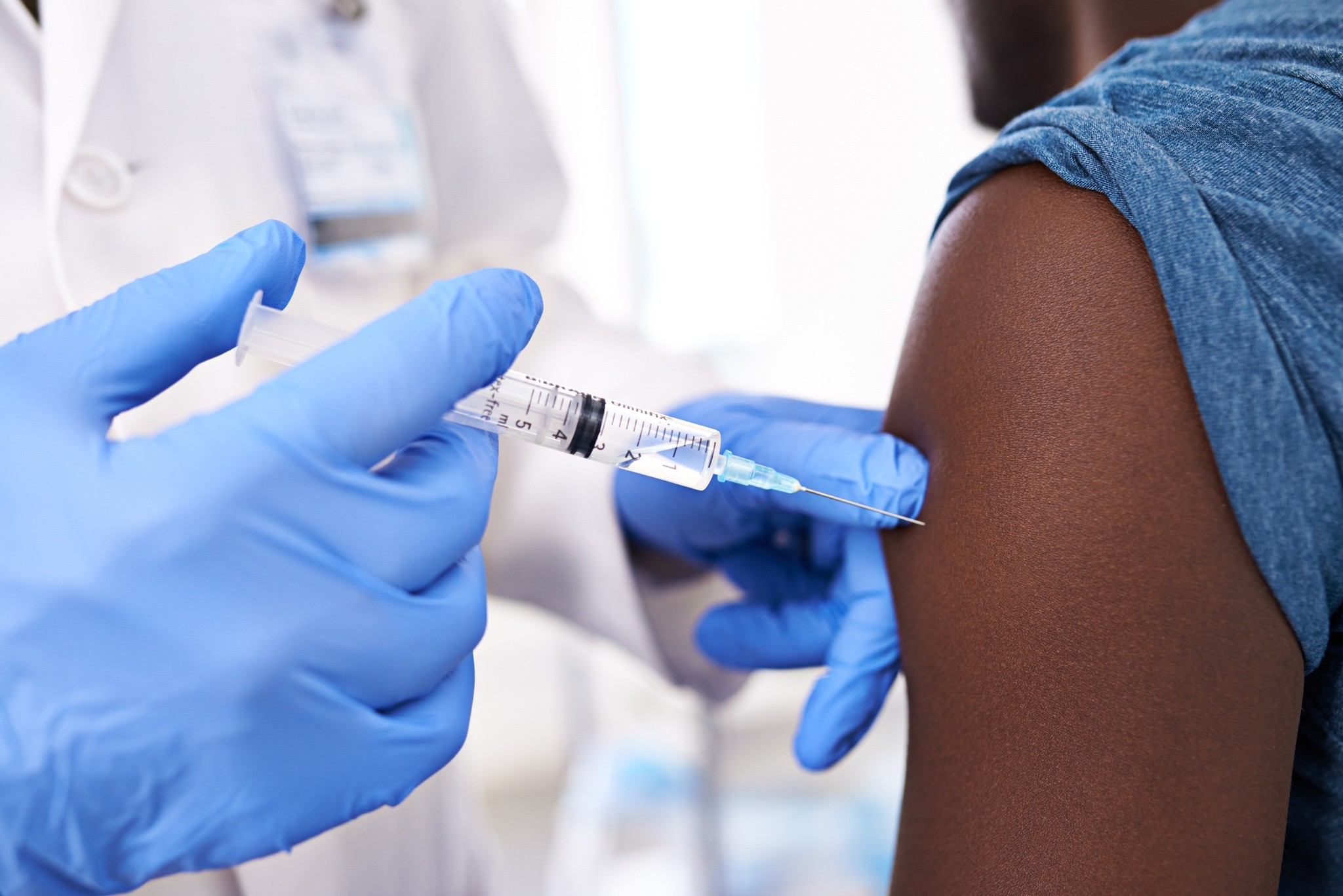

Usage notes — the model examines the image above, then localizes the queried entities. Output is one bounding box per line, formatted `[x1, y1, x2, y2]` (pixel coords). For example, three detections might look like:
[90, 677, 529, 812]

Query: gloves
[614, 385, 934, 774]
[0, 218, 544, 896]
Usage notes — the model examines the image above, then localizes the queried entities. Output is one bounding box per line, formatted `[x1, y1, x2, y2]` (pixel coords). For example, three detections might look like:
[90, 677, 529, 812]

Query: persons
[874, 0, 1343, 895]
[0, 0, 934, 896]
